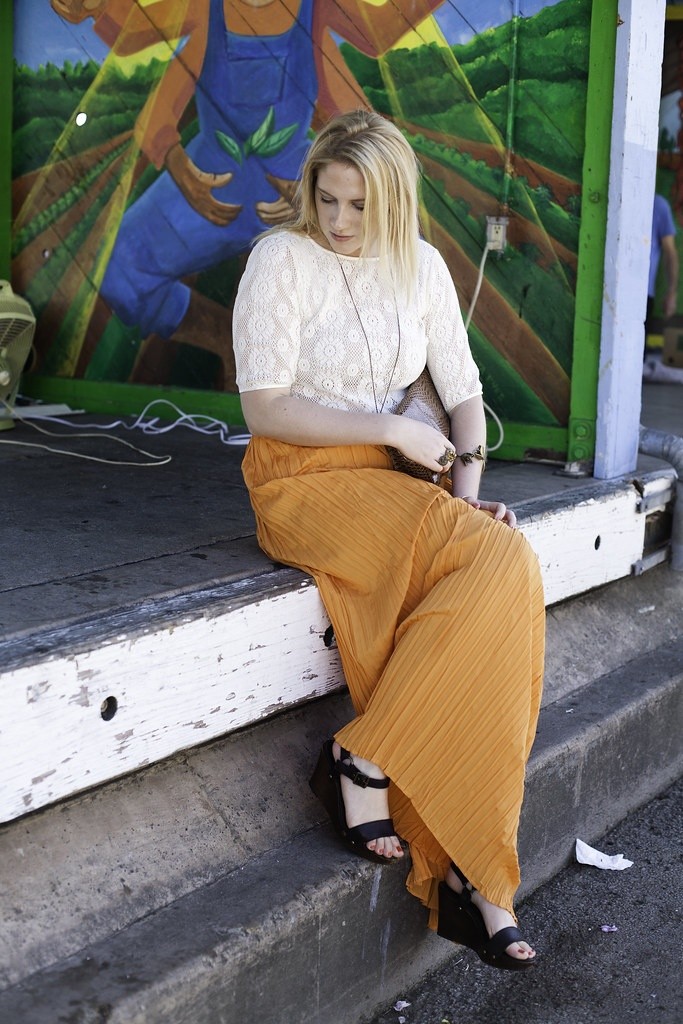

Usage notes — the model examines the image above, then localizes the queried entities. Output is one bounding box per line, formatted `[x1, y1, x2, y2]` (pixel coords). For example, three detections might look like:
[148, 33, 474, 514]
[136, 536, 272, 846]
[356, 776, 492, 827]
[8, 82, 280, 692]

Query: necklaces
[322, 232, 400, 413]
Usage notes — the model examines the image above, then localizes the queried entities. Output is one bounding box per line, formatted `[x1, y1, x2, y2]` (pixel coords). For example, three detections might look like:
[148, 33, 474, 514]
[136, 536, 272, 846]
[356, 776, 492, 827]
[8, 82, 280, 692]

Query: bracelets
[456, 445, 487, 471]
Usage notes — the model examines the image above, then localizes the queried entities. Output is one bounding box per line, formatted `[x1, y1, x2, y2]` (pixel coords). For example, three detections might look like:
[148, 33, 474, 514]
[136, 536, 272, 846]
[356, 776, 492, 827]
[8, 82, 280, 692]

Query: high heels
[437, 861, 534, 972]
[308, 737, 403, 864]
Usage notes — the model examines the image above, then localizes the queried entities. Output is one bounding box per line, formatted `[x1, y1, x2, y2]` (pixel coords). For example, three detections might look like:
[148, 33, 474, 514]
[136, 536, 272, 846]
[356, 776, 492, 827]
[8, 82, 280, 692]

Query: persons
[232, 109, 546, 970]
[644, 192, 677, 363]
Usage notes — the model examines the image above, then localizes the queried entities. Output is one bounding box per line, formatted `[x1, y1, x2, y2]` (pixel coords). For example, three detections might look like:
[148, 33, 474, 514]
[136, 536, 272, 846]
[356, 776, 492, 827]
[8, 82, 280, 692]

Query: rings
[438, 456, 449, 466]
[445, 449, 457, 462]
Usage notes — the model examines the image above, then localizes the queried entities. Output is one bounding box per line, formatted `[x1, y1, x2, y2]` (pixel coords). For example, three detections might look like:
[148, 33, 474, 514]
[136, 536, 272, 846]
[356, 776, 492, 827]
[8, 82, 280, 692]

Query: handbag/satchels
[387, 367, 453, 487]
